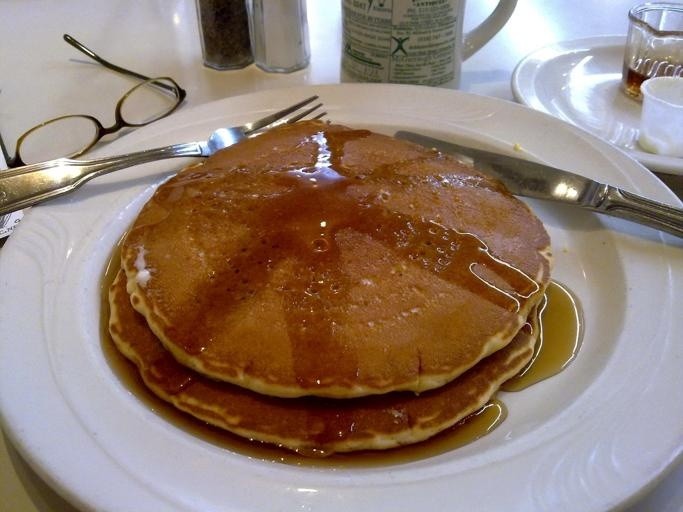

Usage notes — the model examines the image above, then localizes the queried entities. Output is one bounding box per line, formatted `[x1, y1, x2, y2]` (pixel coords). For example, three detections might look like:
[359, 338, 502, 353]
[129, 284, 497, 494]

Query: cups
[634, 74, 683, 161]
[617, 3, 683, 100]
[339, 0, 517, 91]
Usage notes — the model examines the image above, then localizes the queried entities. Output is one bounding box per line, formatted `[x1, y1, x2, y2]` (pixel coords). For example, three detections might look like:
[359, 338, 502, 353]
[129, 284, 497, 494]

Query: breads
[103, 117, 553, 457]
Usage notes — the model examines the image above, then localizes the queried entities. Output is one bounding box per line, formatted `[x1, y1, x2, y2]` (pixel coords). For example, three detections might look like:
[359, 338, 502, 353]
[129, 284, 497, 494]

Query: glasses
[1, 34, 186, 173]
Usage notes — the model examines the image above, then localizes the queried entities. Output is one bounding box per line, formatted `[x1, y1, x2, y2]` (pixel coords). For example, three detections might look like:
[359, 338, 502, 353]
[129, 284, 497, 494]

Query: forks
[0, 92, 329, 215]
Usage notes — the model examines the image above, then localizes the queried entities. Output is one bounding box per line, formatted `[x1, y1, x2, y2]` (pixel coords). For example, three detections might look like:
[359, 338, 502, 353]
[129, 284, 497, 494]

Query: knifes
[391, 128, 682, 243]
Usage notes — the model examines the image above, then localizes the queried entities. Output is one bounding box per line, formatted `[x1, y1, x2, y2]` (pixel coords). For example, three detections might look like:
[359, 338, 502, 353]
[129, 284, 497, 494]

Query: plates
[0, 81, 682, 511]
[509, 35, 683, 176]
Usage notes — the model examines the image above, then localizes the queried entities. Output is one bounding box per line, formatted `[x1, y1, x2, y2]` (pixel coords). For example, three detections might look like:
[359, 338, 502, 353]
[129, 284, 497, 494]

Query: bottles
[194, 0, 311, 75]
